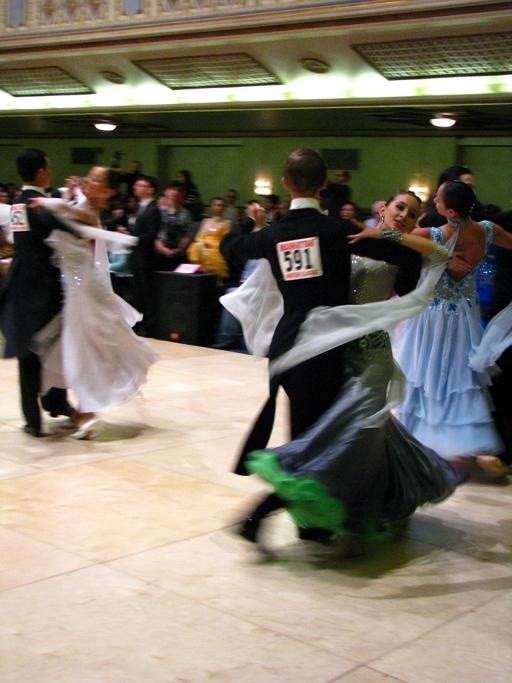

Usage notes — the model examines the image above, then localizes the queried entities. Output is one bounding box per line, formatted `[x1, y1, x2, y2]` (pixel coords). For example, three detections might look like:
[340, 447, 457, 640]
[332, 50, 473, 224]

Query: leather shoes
[43, 398, 78, 418]
[24, 425, 40, 437]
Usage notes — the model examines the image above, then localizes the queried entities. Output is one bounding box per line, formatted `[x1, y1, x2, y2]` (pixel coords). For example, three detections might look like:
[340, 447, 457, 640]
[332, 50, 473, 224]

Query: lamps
[429, 111, 457, 127]
[93, 116, 116, 130]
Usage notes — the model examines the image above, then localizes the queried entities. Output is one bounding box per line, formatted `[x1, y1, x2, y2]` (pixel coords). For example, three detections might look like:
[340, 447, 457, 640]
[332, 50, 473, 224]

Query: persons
[157, 180, 194, 256]
[263, 195, 280, 221]
[419, 163, 511, 462]
[177, 171, 206, 217]
[4, 147, 73, 438]
[247, 191, 461, 542]
[128, 178, 158, 340]
[367, 199, 388, 227]
[339, 202, 357, 221]
[29, 166, 158, 439]
[220, 187, 240, 223]
[220, 181, 512, 481]
[323, 169, 351, 210]
[219, 145, 422, 543]
[186, 197, 232, 278]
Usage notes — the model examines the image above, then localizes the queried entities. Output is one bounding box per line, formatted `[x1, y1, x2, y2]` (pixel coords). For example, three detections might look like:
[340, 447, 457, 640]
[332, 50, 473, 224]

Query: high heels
[72, 418, 103, 440]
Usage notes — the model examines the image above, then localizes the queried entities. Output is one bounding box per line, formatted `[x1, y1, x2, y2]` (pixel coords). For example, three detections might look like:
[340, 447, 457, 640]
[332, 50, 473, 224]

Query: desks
[155, 267, 222, 344]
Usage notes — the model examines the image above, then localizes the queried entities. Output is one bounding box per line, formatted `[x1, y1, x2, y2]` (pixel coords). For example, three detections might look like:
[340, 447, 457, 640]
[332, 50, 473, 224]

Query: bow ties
[45, 188, 52, 193]
